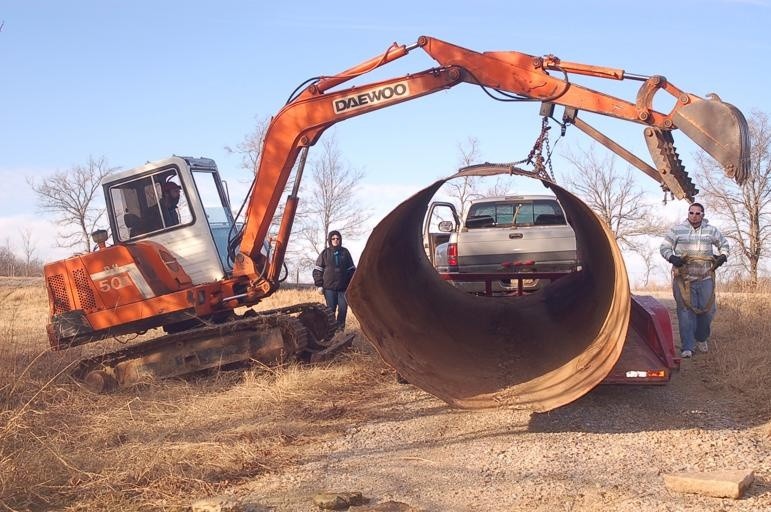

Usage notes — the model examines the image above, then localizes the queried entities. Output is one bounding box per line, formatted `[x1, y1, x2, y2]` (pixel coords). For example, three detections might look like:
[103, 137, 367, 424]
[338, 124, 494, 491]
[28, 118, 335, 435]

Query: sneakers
[681, 340, 708, 358]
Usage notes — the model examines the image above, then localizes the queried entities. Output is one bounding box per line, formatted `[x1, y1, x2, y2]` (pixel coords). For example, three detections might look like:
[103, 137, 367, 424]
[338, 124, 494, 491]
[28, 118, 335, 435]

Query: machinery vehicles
[41, 34, 749, 395]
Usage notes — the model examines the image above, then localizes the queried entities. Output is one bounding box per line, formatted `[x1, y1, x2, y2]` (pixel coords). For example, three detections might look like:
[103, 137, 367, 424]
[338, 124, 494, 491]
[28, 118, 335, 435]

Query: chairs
[534, 213, 563, 226]
[468, 214, 495, 229]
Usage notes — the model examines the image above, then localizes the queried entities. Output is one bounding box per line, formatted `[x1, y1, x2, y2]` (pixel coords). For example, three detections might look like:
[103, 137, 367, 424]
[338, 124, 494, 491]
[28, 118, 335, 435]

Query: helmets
[162, 182, 181, 192]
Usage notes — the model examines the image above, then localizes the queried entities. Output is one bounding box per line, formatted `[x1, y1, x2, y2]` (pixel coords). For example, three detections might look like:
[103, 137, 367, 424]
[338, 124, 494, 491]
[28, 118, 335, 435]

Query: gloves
[710, 254, 727, 271]
[317, 286, 324, 295]
[669, 255, 683, 266]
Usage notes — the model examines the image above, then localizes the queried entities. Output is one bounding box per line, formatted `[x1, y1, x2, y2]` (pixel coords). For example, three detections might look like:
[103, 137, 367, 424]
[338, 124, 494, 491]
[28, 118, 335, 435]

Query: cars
[424, 195, 580, 296]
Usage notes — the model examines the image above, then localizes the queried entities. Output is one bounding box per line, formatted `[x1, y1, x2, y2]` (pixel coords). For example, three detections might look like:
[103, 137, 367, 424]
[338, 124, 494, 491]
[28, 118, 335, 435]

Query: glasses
[331, 237, 340, 240]
[688, 211, 703, 215]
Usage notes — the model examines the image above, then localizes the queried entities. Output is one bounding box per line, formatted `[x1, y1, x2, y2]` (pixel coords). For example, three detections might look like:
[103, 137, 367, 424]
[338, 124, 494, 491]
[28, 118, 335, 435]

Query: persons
[131, 182, 182, 237]
[313, 231, 356, 334]
[660, 202, 729, 359]
